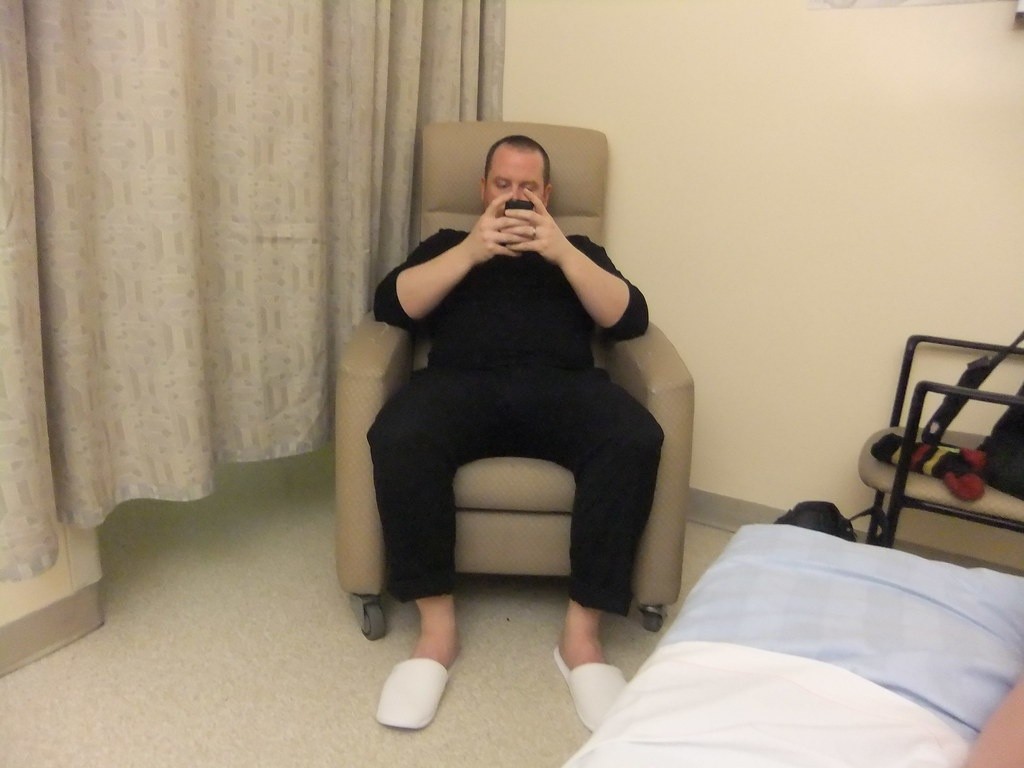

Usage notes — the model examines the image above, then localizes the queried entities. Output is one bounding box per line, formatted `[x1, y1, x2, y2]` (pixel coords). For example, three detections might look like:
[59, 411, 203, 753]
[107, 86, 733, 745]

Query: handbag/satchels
[776, 502, 891, 548]
[975, 383, 1024, 502]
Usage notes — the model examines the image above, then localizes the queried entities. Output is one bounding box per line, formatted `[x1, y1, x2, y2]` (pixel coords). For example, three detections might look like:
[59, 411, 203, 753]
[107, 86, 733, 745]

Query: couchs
[335, 119, 695, 639]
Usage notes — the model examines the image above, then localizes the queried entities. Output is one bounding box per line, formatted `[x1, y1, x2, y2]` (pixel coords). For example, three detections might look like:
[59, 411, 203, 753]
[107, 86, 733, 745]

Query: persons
[365, 134, 666, 734]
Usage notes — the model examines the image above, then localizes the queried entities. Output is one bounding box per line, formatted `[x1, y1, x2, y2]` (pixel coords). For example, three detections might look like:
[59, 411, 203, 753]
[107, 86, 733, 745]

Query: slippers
[552, 641, 626, 734]
[376, 642, 460, 728]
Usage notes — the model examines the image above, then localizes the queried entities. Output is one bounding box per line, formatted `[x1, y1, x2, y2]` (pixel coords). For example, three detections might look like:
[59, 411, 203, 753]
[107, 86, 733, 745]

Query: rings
[533, 225, 537, 239]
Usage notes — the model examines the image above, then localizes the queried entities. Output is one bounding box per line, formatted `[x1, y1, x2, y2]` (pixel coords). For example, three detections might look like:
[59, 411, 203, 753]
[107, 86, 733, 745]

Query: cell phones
[504, 200, 534, 217]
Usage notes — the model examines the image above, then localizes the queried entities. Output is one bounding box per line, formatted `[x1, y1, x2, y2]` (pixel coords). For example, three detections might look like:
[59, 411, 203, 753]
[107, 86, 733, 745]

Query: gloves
[871, 431, 987, 503]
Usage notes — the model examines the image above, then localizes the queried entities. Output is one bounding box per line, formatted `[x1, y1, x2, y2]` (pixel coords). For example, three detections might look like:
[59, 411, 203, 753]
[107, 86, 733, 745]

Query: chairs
[858, 333, 1024, 578]
